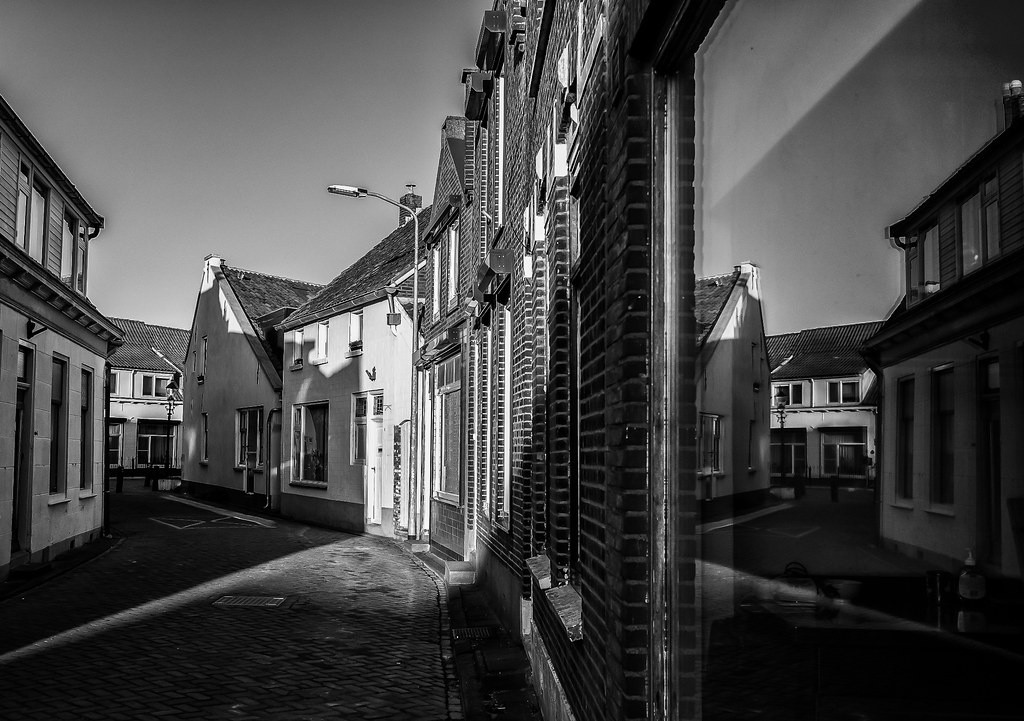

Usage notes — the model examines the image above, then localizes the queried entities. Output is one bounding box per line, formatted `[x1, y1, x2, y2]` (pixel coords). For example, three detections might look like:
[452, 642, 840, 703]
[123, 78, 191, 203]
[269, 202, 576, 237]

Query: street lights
[327, 184, 420, 543]
